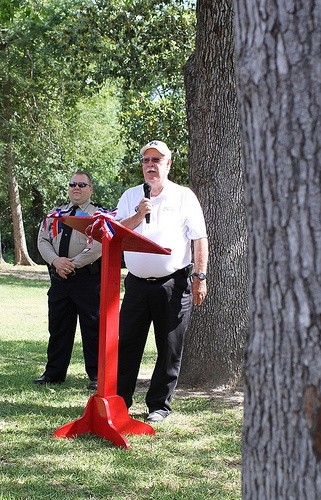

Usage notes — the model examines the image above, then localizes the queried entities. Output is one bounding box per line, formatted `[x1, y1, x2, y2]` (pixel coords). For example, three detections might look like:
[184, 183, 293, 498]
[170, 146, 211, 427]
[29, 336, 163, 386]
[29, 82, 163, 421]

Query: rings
[64, 268, 67, 271]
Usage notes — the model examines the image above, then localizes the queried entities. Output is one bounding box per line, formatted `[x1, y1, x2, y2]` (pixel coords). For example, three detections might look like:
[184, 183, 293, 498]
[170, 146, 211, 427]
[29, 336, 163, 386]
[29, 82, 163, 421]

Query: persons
[34, 171, 102, 390]
[115, 140, 209, 421]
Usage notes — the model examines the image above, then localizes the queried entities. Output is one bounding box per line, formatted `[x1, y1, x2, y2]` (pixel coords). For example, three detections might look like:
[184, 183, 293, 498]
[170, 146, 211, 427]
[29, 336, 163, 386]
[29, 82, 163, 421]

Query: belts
[128, 270, 182, 283]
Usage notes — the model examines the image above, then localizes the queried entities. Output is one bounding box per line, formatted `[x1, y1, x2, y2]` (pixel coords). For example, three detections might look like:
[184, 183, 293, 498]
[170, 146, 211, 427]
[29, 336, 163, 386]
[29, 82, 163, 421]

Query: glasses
[69, 181, 91, 188]
[142, 156, 166, 163]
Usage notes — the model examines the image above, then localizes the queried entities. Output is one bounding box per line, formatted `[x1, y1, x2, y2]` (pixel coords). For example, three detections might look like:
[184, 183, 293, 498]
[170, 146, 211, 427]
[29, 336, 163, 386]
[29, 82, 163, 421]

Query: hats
[140, 140, 171, 160]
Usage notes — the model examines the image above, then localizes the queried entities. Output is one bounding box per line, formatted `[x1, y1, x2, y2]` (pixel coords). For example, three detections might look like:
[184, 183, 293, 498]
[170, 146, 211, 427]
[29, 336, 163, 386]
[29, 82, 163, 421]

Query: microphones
[143, 182, 152, 223]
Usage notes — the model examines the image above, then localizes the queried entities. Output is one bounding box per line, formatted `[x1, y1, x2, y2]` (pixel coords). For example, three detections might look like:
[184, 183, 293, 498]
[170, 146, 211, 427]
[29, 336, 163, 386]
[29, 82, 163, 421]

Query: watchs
[192, 273, 207, 280]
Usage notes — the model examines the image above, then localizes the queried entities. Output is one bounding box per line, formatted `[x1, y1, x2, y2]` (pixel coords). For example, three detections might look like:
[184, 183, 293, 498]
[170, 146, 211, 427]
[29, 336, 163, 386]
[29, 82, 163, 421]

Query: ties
[58, 206, 79, 258]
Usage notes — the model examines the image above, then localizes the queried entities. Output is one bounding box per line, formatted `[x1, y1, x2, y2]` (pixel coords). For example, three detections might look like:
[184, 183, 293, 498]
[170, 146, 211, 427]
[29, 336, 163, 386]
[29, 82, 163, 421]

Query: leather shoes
[89, 382, 97, 390]
[33, 375, 56, 384]
[147, 412, 164, 422]
